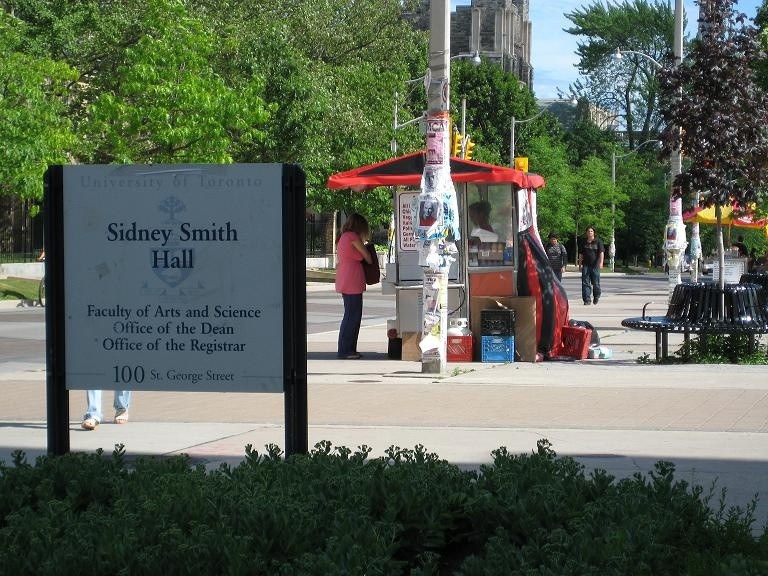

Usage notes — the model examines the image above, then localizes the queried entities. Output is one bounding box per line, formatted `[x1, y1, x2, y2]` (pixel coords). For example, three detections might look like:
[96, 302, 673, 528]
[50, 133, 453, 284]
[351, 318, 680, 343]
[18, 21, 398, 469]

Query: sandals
[114, 409, 128, 424]
[81, 418, 96, 430]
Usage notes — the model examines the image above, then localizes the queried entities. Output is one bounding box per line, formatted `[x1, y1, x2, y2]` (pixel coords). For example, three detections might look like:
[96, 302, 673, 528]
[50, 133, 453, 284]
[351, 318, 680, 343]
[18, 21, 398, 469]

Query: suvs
[703, 249, 732, 275]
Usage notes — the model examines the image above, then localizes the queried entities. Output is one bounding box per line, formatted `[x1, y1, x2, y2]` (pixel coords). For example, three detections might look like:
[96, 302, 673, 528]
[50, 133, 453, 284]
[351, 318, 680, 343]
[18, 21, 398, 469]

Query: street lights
[390, 51, 481, 157]
[610, 140, 663, 269]
[510, 97, 577, 168]
[614, 46, 703, 283]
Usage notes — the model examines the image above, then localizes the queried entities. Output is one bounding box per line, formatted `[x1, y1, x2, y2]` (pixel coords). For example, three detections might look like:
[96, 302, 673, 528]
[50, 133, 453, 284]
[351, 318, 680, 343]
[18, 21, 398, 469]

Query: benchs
[622, 283, 768, 362]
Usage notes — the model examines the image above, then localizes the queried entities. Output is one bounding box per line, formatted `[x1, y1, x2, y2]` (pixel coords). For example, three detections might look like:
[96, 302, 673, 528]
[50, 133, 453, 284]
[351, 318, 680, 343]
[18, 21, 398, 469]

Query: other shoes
[336, 352, 360, 359]
[584, 300, 591, 305]
[593, 296, 598, 305]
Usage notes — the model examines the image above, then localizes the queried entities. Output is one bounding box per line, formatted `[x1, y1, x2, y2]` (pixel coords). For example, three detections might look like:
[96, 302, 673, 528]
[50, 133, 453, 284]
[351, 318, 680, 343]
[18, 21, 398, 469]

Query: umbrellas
[682, 191, 768, 249]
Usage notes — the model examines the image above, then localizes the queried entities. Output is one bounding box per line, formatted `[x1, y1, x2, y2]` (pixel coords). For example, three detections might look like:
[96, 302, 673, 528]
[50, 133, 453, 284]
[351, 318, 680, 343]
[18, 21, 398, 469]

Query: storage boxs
[447, 336, 474, 363]
[480, 336, 514, 363]
[402, 331, 422, 361]
[562, 326, 592, 360]
[480, 309, 516, 336]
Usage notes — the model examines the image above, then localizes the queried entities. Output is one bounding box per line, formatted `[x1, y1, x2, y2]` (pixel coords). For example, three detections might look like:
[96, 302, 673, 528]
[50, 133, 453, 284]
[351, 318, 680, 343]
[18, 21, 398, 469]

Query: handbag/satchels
[360, 242, 380, 286]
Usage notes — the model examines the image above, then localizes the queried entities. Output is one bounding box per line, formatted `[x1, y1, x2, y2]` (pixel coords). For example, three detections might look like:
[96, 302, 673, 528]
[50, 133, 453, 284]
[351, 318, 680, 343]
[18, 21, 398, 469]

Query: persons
[37, 252, 45, 260]
[335, 215, 372, 360]
[732, 235, 759, 266]
[545, 234, 567, 283]
[419, 202, 436, 226]
[468, 200, 495, 248]
[579, 227, 605, 305]
[82, 391, 131, 429]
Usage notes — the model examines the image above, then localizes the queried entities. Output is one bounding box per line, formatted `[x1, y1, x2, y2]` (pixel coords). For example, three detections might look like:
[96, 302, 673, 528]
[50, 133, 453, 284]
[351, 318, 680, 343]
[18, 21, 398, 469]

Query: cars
[665, 254, 691, 274]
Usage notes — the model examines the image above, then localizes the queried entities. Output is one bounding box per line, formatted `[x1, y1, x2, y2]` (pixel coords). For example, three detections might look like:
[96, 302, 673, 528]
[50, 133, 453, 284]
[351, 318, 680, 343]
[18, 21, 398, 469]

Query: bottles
[498, 242, 504, 260]
[491, 242, 497, 256]
